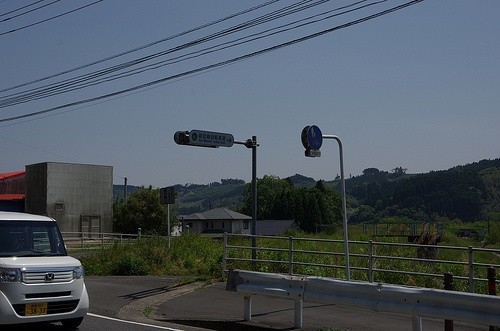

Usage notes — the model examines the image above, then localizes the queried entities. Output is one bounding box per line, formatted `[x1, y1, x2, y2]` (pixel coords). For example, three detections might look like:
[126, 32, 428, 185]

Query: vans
[0, 210, 91, 329]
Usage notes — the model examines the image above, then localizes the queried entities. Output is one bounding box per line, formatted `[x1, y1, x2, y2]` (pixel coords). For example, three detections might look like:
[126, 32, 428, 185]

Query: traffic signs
[187, 130, 234, 148]
